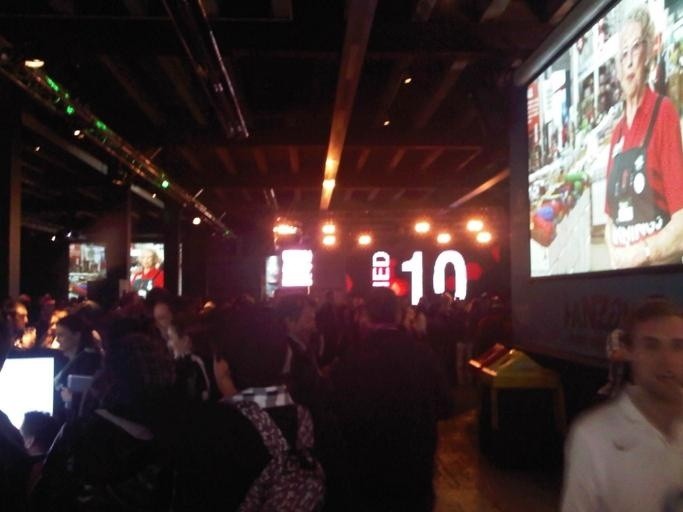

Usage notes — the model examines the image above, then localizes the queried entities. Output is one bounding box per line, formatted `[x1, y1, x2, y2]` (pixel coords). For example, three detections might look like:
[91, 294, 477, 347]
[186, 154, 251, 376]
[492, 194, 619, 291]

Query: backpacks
[217, 395, 326, 512]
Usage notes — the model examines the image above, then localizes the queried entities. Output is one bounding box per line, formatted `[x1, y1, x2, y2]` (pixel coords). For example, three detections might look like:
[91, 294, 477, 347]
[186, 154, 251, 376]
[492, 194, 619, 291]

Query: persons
[593, 5, 681, 272]
[560, 295, 681, 511]
[2, 273, 509, 512]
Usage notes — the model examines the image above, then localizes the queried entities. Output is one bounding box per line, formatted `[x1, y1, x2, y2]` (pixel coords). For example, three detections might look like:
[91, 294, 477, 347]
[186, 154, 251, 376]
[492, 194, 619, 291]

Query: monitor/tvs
[1, 349, 63, 430]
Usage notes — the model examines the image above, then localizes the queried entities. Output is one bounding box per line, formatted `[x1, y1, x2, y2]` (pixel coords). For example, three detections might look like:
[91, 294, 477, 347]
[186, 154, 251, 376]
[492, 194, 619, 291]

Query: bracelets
[641, 240, 651, 267]
